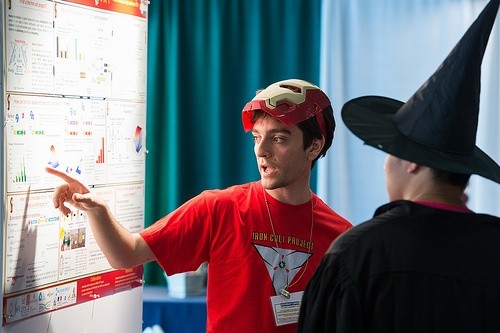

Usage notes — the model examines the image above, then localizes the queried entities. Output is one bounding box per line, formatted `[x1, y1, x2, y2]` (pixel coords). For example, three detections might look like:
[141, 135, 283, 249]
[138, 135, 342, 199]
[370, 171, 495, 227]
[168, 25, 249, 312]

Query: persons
[43, 78, 355, 333]
[297, 1, 500, 333]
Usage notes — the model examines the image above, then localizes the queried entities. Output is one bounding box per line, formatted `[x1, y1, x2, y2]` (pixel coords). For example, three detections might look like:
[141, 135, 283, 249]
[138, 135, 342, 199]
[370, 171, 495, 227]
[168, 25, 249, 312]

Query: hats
[341, 1, 499, 185]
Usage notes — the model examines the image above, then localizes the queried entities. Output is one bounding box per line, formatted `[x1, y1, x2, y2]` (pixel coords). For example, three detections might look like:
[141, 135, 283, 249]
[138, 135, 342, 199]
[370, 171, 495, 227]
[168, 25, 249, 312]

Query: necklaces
[262, 184, 314, 298]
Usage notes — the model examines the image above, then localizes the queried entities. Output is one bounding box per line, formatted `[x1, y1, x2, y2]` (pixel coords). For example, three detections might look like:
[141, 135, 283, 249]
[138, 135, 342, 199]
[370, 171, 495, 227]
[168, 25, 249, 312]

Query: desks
[142, 284, 207, 333]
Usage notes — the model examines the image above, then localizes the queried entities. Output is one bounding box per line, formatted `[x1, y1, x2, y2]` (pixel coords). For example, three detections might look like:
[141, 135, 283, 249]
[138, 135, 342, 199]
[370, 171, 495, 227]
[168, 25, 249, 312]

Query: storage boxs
[164, 268, 206, 298]
